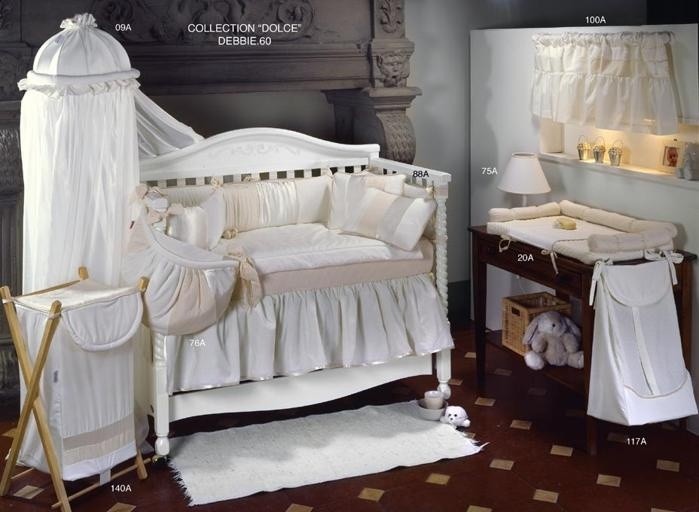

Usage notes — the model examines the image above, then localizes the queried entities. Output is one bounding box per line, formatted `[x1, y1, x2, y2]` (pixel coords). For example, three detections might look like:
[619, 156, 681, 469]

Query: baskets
[502, 291, 574, 358]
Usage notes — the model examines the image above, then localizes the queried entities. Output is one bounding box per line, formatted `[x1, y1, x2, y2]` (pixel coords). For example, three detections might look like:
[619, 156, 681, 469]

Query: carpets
[164, 396, 489, 509]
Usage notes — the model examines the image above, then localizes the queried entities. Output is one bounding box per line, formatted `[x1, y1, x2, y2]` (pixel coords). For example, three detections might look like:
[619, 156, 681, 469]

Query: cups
[592, 143, 605, 163]
[607, 147, 622, 166]
[576, 143, 590, 160]
[423, 390, 444, 409]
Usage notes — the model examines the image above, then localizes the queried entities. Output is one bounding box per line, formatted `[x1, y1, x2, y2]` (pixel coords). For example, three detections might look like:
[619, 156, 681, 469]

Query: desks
[468, 222, 697, 458]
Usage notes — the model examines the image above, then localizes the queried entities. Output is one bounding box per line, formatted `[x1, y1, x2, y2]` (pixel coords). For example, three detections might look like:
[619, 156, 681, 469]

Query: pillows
[165, 189, 227, 253]
[327, 172, 437, 252]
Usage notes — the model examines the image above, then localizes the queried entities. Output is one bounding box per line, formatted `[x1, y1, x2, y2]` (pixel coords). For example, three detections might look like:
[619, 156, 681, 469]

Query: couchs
[140, 126, 453, 470]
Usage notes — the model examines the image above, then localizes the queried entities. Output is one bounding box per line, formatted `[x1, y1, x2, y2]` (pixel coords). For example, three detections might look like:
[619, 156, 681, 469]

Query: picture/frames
[656, 138, 688, 176]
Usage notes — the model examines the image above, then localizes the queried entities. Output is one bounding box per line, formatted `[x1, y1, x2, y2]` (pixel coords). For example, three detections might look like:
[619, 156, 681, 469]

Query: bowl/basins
[415, 398, 449, 421]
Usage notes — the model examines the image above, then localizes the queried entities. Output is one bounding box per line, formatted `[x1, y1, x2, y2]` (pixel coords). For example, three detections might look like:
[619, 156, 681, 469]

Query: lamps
[496, 152, 551, 208]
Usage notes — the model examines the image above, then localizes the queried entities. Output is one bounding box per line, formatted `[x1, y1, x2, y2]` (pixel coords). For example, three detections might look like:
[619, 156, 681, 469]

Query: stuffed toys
[439, 405, 471, 428]
[521, 309, 584, 370]
[677, 145, 699, 180]
